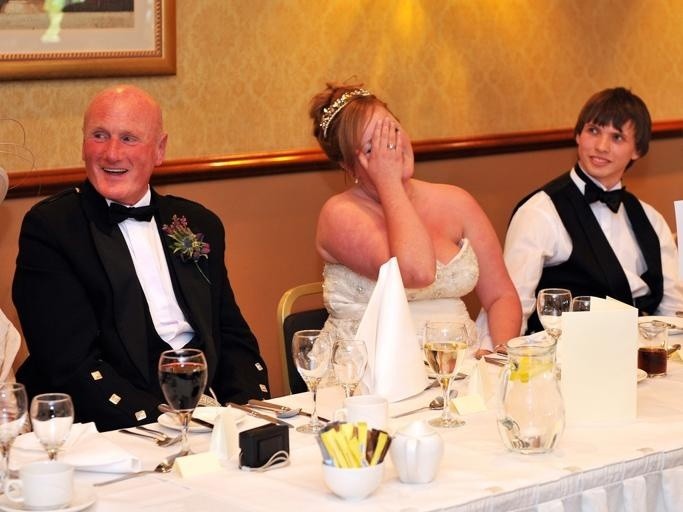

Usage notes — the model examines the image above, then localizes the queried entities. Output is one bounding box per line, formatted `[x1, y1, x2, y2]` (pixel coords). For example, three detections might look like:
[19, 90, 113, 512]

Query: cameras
[238, 423, 289, 471]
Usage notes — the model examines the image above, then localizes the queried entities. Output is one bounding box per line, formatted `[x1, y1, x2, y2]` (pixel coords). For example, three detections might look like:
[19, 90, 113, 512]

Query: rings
[388, 144, 398, 153]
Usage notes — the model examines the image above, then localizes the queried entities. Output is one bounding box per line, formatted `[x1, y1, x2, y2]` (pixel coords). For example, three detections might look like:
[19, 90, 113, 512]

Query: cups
[637, 322, 667, 376]
[496, 338, 563, 455]
[5, 461, 75, 510]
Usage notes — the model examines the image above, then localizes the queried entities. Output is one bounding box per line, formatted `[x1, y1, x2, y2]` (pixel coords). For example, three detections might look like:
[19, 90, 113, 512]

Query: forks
[118, 429, 183, 448]
[136, 426, 182, 443]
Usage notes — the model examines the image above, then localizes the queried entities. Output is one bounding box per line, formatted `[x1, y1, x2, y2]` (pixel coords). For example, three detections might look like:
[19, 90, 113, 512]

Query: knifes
[248, 398, 343, 423]
[226, 402, 294, 429]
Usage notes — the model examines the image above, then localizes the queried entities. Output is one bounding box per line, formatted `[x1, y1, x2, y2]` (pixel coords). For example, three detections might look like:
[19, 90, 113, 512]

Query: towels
[9, 421, 165, 474]
[351, 258, 433, 401]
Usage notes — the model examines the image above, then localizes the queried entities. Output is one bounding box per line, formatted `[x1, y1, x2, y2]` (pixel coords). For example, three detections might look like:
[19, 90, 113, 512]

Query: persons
[311, 82, 522, 390]
[470, 88, 681, 363]
[10, 84, 270, 430]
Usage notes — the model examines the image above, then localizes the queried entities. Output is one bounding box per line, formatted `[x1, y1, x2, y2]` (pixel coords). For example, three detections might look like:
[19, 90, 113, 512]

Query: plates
[0, 484, 98, 511]
[634, 369, 648, 383]
[158, 406, 231, 433]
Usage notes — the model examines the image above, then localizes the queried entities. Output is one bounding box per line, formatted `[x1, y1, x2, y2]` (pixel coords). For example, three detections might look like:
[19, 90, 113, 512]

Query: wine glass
[423, 324, 465, 428]
[0, 383, 28, 492]
[288, 330, 332, 436]
[331, 340, 368, 423]
[158, 349, 210, 464]
[31, 393, 74, 481]
[570, 296, 595, 320]
[536, 289, 572, 376]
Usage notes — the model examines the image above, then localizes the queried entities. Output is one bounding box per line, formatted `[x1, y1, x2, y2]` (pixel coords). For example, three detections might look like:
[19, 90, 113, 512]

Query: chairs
[275, 280, 332, 395]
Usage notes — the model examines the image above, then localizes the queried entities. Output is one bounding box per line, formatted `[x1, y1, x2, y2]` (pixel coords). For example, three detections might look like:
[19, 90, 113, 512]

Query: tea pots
[389, 420, 442, 484]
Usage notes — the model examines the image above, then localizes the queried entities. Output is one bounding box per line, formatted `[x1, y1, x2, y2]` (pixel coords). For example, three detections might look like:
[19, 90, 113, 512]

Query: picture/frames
[0, 0, 176, 80]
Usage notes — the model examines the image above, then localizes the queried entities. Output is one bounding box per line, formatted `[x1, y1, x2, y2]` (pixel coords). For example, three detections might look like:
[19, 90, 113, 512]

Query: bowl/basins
[321, 462, 382, 501]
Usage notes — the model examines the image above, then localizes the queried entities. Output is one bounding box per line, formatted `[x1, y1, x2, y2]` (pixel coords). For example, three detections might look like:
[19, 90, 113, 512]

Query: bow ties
[109, 203, 154, 222]
[573, 161, 627, 214]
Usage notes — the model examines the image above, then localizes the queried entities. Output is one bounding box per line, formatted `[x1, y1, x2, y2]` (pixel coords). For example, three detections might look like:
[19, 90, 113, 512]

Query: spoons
[387, 396, 445, 421]
